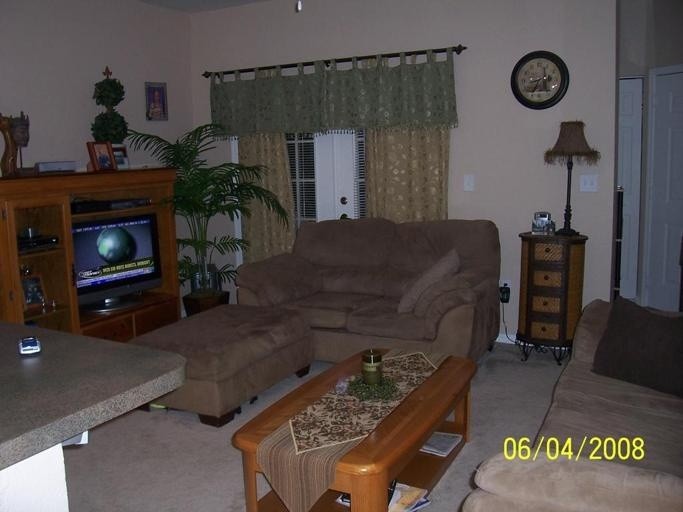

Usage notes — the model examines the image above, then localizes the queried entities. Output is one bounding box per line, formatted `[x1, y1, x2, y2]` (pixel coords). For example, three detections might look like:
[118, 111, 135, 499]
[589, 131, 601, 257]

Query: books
[335, 480, 431, 511]
[419, 431, 463, 458]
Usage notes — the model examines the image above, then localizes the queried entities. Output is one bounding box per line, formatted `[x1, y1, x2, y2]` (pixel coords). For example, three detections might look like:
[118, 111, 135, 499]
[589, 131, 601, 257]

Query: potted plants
[120, 124, 291, 317]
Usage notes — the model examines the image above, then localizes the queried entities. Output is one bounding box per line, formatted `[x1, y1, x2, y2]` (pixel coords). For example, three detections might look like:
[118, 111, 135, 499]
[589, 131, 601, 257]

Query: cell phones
[18, 336, 41, 354]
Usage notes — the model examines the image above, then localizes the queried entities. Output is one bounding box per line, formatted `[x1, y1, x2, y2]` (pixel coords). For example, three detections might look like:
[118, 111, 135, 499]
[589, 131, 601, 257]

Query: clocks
[511, 51, 569, 111]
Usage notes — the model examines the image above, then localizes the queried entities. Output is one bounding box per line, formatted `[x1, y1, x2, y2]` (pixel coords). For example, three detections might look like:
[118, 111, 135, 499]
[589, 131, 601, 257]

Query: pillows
[591, 294, 682, 398]
[396, 247, 460, 315]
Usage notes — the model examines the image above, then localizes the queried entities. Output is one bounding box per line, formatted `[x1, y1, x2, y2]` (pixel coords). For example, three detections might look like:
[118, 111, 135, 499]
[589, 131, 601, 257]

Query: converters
[500, 287, 510, 303]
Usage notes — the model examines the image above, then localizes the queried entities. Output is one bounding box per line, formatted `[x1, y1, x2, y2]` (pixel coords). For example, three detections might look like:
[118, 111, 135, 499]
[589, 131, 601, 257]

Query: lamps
[542, 121, 602, 237]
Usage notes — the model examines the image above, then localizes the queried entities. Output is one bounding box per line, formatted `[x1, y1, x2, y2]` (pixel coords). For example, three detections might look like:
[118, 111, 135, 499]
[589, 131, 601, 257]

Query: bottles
[360, 348, 383, 387]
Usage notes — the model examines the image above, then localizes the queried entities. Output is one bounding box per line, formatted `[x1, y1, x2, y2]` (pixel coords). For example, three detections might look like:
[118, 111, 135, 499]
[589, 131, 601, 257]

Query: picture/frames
[86, 140, 117, 171]
[19, 272, 50, 312]
[143, 82, 168, 122]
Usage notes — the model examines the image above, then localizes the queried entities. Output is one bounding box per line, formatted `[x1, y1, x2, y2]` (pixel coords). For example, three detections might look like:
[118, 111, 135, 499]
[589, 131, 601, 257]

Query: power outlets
[497, 279, 511, 293]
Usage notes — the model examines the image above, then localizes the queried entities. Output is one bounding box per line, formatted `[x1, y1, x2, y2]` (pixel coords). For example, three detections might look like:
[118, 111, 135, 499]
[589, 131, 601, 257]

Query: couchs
[129, 303, 314, 428]
[233, 216, 500, 366]
[458, 295, 682, 511]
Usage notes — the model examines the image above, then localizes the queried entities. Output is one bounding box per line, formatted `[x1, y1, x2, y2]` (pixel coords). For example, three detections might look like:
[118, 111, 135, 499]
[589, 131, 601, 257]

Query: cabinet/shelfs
[515, 231, 589, 365]
[0, 166, 183, 344]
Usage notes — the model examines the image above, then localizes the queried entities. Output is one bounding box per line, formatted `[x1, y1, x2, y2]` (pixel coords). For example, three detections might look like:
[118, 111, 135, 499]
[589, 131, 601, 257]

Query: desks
[1, 322, 188, 511]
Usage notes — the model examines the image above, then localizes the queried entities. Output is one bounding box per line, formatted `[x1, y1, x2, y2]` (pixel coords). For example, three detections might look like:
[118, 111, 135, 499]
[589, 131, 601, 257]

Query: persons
[9, 116, 29, 147]
[147, 89, 164, 118]
[97, 150, 111, 169]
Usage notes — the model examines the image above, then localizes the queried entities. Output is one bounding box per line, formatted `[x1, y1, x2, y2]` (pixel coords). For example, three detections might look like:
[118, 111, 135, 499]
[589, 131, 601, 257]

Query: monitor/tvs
[72, 213, 162, 312]
